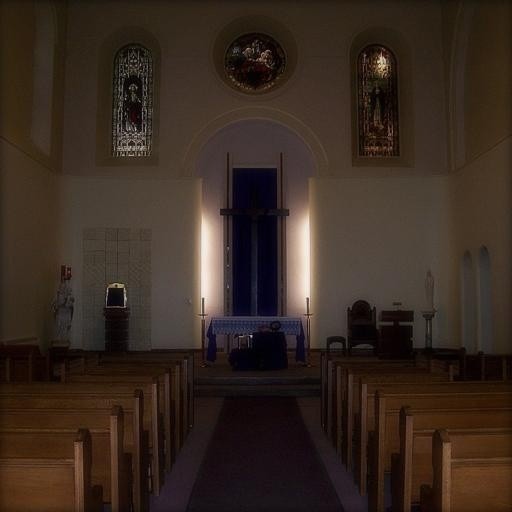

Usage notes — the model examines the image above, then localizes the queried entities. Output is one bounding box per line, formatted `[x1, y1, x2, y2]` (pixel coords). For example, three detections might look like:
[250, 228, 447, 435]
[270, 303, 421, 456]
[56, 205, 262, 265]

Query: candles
[306, 297, 310, 314]
[202, 297, 204, 314]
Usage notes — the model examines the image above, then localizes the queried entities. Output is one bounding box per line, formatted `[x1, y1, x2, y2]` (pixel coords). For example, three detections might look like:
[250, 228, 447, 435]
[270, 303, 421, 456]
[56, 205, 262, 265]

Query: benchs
[319, 353, 512, 512]
[0, 351, 194, 511]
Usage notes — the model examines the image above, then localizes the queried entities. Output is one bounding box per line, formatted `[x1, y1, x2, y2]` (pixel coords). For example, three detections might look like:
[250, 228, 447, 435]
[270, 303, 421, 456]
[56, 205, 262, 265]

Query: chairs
[346, 299, 379, 353]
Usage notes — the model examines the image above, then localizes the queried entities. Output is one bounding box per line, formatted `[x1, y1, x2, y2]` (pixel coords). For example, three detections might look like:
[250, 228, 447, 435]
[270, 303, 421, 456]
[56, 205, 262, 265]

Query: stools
[325, 335, 347, 351]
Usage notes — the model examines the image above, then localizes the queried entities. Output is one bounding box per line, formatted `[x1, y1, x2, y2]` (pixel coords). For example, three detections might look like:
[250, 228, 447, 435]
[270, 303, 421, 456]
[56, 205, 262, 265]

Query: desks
[205, 314, 307, 373]
